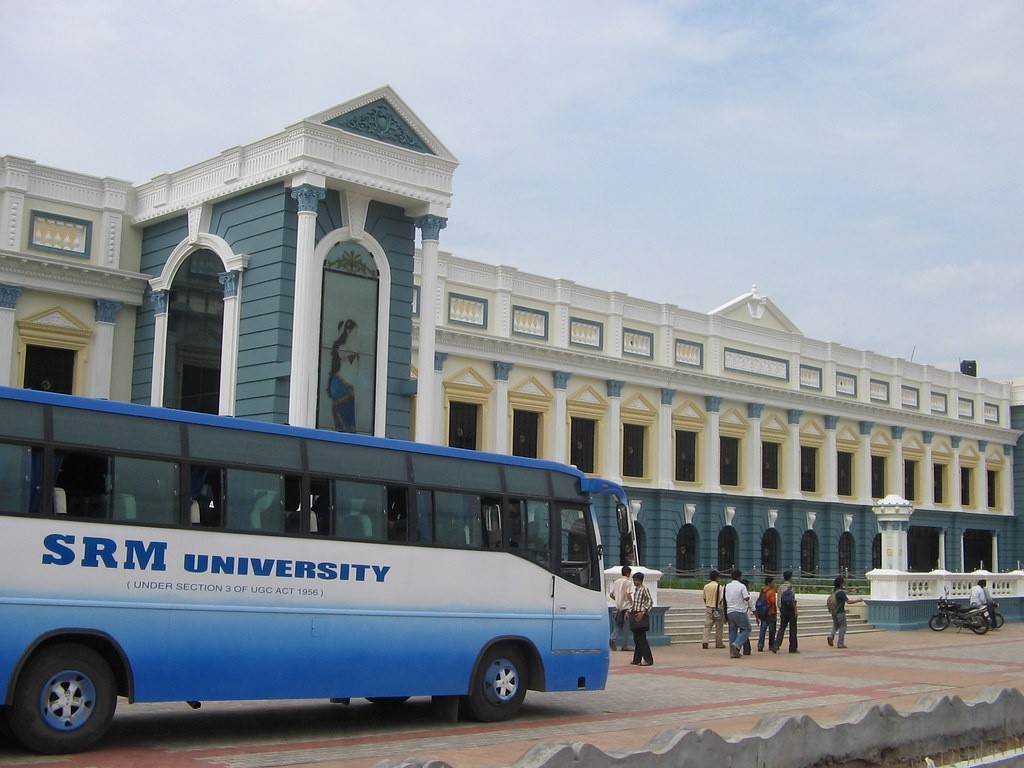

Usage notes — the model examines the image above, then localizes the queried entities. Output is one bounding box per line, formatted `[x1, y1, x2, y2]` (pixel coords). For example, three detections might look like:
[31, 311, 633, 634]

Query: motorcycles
[929, 596, 1004, 635]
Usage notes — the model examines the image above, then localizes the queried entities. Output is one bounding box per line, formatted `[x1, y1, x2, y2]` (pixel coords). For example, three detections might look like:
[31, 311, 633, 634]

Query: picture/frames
[316, 268, 379, 437]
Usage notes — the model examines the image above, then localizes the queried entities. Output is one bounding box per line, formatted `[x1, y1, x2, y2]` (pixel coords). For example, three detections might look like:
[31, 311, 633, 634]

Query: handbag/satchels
[712, 608, 722, 622]
[629, 614, 649, 632]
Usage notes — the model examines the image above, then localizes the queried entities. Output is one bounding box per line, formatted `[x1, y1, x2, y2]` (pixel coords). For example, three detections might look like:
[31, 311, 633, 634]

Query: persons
[757, 577, 779, 652]
[701, 570, 725, 648]
[740, 579, 760, 656]
[983, 580, 1000, 628]
[968, 580, 994, 631]
[769, 571, 799, 654]
[827, 576, 864, 648]
[720, 570, 752, 658]
[624, 572, 654, 666]
[609, 565, 634, 652]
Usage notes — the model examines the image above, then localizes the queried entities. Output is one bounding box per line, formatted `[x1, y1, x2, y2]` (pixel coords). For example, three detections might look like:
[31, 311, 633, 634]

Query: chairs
[53, 488, 470, 547]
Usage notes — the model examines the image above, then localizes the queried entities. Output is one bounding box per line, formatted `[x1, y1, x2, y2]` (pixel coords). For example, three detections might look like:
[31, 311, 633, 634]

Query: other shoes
[639, 660, 653, 666]
[827, 637, 833, 646]
[770, 648, 771, 649]
[703, 643, 708, 649]
[789, 650, 798, 653]
[621, 648, 633, 651]
[630, 661, 641, 664]
[609, 639, 617, 651]
[758, 646, 763, 651]
[772, 648, 776, 653]
[730, 642, 740, 654]
[838, 644, 847, 648]
[716, 645, 725, 648]
[731, 654, 741, 658]
[744, 652, 751, 655]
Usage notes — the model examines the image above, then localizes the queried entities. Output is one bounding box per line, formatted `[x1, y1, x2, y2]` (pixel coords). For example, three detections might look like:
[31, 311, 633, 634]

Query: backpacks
[755, 590, 772, 620]
[827, 588, 843, 617]
[781, 587, 795, 617]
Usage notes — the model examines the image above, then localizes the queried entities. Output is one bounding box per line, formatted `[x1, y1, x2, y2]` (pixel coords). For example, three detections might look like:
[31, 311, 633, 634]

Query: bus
[0, 381, 633, 758]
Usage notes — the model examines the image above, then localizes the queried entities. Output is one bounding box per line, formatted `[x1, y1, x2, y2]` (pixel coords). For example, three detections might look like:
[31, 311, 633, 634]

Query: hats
[709, 571, 720, 579]
